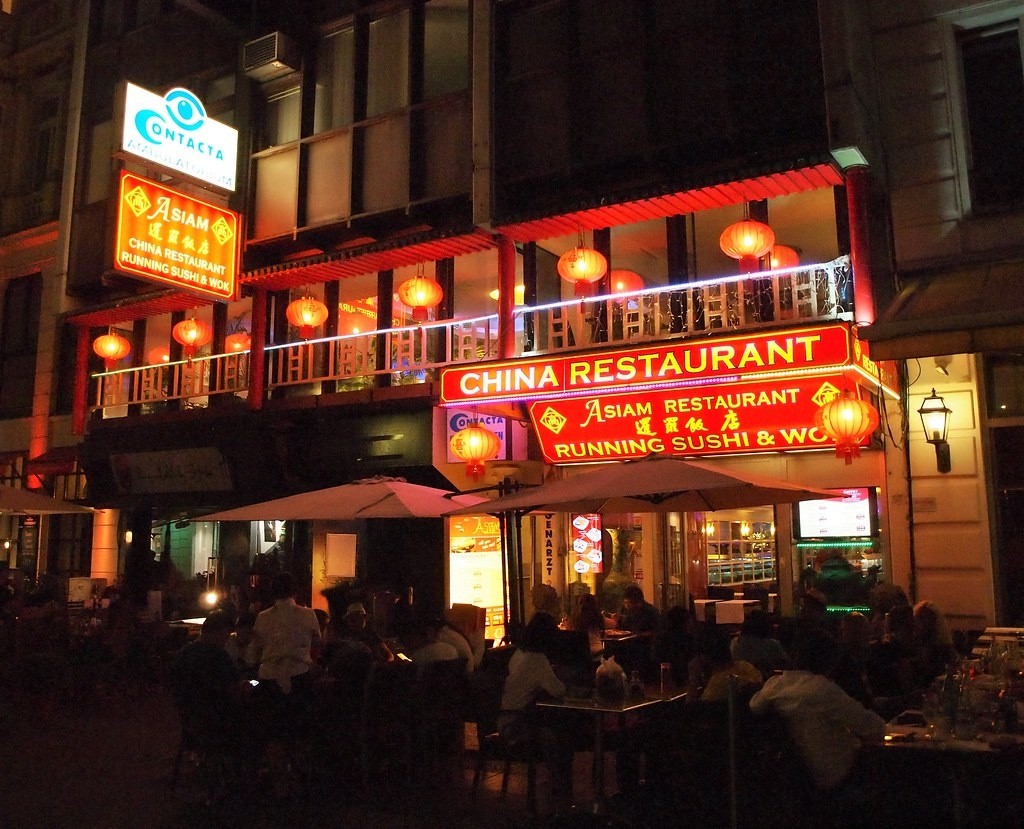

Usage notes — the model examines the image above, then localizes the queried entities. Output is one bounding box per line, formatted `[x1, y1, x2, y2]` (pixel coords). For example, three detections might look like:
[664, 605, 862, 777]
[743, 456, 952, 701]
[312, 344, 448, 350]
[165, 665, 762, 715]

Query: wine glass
[921, 634, 1024, 746]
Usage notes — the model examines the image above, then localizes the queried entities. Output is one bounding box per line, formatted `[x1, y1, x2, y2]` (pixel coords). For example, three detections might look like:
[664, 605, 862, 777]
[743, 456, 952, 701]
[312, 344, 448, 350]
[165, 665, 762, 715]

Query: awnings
[27, 443, 79, 473]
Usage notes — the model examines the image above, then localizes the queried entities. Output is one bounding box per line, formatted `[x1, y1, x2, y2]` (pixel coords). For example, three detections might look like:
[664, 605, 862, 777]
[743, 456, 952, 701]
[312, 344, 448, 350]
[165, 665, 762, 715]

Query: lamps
[916, 386, 953, 454]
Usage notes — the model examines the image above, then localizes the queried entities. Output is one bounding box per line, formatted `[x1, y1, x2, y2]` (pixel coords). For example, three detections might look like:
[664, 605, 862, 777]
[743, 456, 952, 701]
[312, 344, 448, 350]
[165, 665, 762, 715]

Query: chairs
[467, 669, 562, 810]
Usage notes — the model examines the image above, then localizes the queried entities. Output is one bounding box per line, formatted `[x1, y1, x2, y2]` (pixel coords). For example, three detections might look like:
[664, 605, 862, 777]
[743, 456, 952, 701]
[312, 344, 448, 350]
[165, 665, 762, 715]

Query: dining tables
[533, 687, 719, 827]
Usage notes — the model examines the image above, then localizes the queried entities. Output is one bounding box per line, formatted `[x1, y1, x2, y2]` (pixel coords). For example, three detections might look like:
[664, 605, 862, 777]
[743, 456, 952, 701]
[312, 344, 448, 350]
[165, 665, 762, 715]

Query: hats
[341, 602, 370, 619]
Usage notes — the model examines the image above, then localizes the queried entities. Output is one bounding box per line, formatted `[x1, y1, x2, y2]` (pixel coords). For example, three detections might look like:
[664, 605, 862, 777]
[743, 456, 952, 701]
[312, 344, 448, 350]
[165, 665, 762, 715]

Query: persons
[750, 628, 888, 802]
[499, 611, 576, 805]
[171, 611, 251, 745]
[256, 571, 324, 805]
[2, 561, 62, 658]
[314, 585, 962, 706]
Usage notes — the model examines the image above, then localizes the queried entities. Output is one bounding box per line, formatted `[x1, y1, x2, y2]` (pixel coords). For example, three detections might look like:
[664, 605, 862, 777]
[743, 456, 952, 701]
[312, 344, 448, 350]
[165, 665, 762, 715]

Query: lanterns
[287, 295, 329, 338]
[398, 277, 444, 340]
[148, 346, 170, 365]
[224, 333, 251, 356]
[720, 218, 775, 295]
[817, 390, 880, 464]
[559, 246, 607, 317]
[91, 333, 132, 369]
[763, 244, 799, 268]
[451, 422, 500, 482]
[172, 317, 213, 368]
[605, 270, 644, 293]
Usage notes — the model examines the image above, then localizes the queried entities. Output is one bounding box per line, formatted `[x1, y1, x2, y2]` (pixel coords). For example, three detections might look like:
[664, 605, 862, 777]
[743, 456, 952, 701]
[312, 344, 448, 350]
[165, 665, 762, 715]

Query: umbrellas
[441, 454, 849, 507]
[188, 474, 488, 520]
[0, 480, 91, 518]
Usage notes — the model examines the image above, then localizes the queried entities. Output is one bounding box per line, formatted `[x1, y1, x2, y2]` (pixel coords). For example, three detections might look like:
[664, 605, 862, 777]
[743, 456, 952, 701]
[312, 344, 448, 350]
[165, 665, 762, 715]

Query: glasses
[349, 617, 365, 624]
